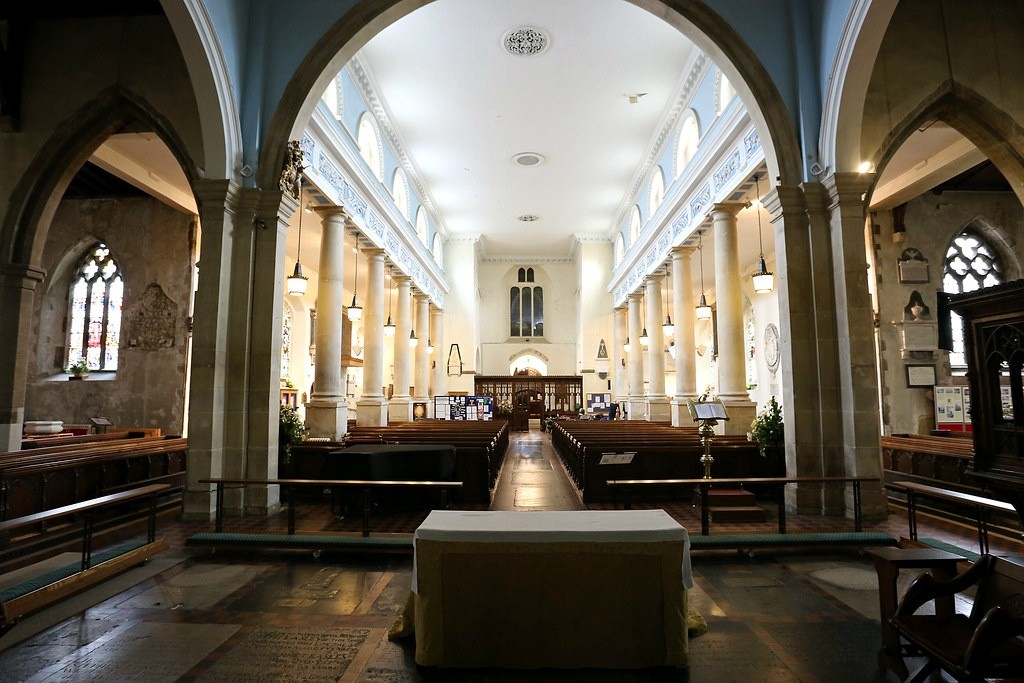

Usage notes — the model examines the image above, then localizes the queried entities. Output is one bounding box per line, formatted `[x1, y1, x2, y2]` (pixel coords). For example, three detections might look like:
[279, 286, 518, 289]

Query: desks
[861, 548, 969, 681]
[388, 509, 709, 672]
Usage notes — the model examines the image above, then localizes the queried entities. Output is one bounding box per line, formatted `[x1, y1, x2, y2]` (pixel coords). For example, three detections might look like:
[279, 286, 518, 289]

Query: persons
[576, 408, 608, 421]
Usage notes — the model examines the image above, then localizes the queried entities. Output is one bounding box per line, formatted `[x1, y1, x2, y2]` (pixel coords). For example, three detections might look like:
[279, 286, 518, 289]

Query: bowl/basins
[24, 421, 64, 435]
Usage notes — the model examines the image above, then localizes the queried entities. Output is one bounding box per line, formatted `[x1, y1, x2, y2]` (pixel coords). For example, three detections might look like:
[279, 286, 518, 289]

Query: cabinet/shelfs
[948, 277, 1024, 491]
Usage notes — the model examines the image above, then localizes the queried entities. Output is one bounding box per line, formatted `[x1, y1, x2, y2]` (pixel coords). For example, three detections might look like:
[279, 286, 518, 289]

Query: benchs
[894, 480, 1016, 573]
[184, 416, 509, 555]
[0, 428, 189, 627]
[551, 419, 897, 556]
[888, 553, 1024, 683]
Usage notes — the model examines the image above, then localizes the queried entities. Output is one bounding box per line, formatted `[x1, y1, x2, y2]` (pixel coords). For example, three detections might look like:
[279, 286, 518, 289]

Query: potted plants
[278, 400, 311, 504]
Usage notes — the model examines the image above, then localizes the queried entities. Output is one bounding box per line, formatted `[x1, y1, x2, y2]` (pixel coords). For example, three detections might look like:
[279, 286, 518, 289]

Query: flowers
[67, 355, 96, 373]
[746, 395, 784, 458]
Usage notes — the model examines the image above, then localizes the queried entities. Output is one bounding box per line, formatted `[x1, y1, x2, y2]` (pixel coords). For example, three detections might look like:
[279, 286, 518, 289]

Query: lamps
[348, 231, 363, 321]
[623, 308, 629, 352]
[428, 304, 434, 354]
[409, 287, 418, 349]
[752, 177, 775, 293]
[287, 179, 308, 297]
[662, 264, 674, 337]
[695, 231, 711, 321]
[639, 285, 649, 347]
[384, 264, 396, 338]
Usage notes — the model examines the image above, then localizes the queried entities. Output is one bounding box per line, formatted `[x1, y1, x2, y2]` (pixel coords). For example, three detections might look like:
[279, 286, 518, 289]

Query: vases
[74, 372, 84, 378]
